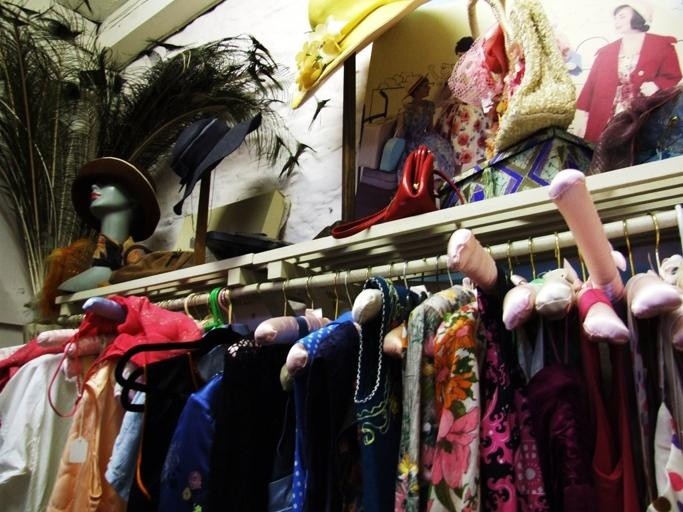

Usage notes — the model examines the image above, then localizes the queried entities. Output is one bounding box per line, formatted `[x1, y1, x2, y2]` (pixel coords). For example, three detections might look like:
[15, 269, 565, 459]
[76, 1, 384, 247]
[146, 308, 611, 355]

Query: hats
[71, 156, 160, 242]
[171, 112, 263, 215]
[402, 71, 429, 101]
[292, 0, 425, 110]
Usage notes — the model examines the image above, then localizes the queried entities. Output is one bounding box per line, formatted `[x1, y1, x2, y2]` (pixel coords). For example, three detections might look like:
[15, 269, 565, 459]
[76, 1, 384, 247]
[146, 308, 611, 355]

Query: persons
[32, 155, 161, 315]
[570, 3, 681, 148]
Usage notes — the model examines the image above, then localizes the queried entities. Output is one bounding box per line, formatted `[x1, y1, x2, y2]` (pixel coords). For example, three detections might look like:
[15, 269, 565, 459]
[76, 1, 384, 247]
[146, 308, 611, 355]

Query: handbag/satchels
[449, 1, 576, 172]
[630, 89, 682, 164]
[332, 146, 464, 239]
[108, 245, 196, 283]
